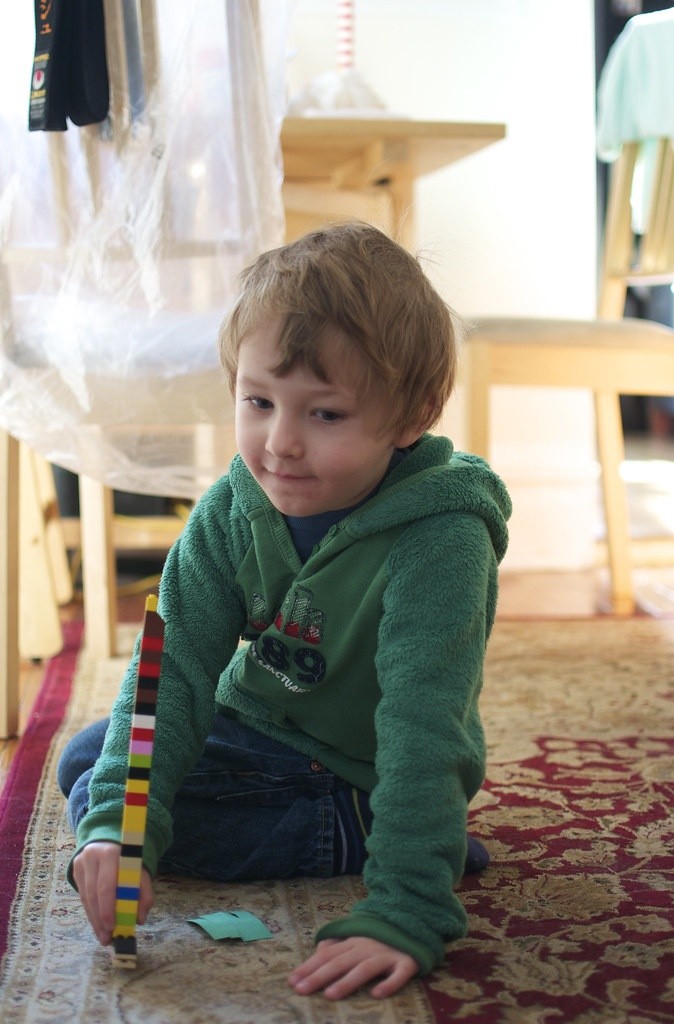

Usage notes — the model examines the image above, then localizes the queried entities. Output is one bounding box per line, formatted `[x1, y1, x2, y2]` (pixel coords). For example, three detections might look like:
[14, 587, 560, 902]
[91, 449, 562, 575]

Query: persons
[56, 220, 512, 999]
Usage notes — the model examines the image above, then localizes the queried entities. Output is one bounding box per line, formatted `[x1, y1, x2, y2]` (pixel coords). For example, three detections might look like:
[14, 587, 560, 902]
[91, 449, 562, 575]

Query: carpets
[0, 611, 674, 1024]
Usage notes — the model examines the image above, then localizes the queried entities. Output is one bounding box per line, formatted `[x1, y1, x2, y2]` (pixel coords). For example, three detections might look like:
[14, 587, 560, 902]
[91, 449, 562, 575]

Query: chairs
[455, 135, 674, 622]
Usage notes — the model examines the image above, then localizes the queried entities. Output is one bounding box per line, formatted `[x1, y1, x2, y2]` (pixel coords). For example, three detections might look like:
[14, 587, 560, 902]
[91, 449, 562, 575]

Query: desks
[78, 104, 509, 662]
